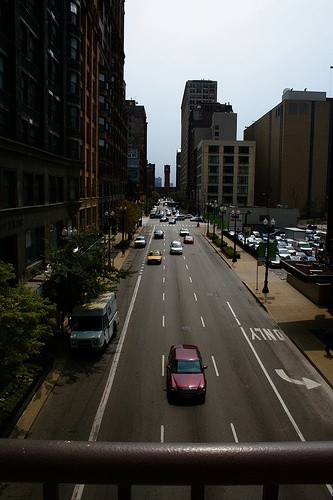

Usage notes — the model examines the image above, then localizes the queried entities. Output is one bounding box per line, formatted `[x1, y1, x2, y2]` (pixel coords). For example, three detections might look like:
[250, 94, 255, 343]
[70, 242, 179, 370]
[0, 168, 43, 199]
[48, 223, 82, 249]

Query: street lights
[104, 210, 115, 272]
[119, 206, 127, 249]
[220, 207, 227, 249]
[205, 202, 212, 235]
[262, 217, 276, 293]
[61, 226, 78, 281]
[191, 187, 200, 227]
[212, 204, 218, 241]
[231, 210, 241, 262]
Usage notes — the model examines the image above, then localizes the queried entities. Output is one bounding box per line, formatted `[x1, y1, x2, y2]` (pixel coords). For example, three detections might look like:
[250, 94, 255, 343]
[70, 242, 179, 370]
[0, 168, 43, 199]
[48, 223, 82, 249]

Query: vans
[69, 293, 119, 354]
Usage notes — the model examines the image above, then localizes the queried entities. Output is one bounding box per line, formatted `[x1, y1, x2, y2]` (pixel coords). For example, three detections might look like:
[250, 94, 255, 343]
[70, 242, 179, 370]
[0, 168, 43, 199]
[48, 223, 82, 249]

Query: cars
[149, 199, 203, 224]
[227, 227, 327, 268]
[147, 250, 163, 265]
[183, 235, 195, 245]
[179, 228, 191, 236]
[169, 241, 184, 255]
[134, 236, 146, 247]
[153, 229, 165, 239]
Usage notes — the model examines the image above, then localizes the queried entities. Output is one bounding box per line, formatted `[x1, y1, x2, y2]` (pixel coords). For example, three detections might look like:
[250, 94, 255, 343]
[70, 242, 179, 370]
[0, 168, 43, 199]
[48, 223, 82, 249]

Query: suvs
[166, 344, 209, 403]
